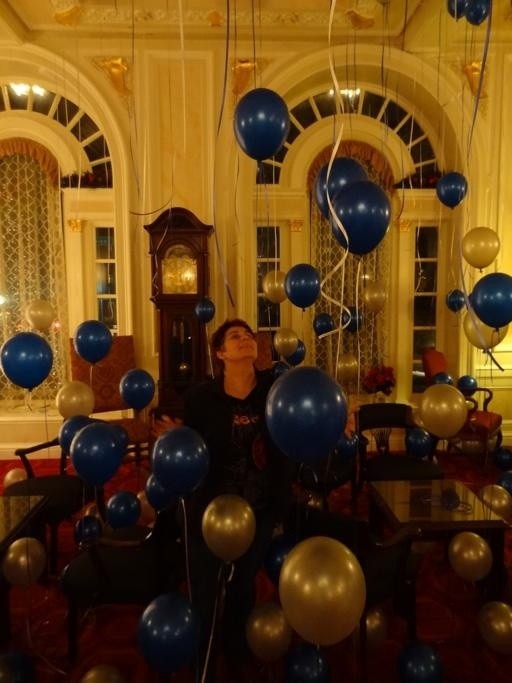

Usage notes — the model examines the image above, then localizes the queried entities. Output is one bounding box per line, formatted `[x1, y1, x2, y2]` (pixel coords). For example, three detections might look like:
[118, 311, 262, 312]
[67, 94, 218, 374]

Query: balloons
[361, 284, 387, 313]
[315, 156, 366, 218]
[328, 180, 391, 255]
[446, 290, 466, 312]
[194, 299, 216, 323]
[463, 227, 499, 269]
[314, 313, 333, 334]
[30, 301, 52, 330]
[262, 272, 289, 304]
[343, 307, 362, 332]
[471, 273, 512, 327]
[233, 87, 290, 161]
[285, 265, 320, 308]
[448, 1, 491, 24]
[438, 172, 469, 208]
[463, 311, 507, 352]
[0, 320, 512, 683]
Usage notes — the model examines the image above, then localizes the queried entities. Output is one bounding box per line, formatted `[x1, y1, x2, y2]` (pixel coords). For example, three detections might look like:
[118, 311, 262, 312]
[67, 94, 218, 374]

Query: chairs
[291, 435, 365, 517]
[61, 498, 181, 668]
[4, 654, 35, 683]
[353, 403, 431, 485]
[443, 389, 504, 469]
[305, 510, 407, 665]
[7, 432, 114, 576]
